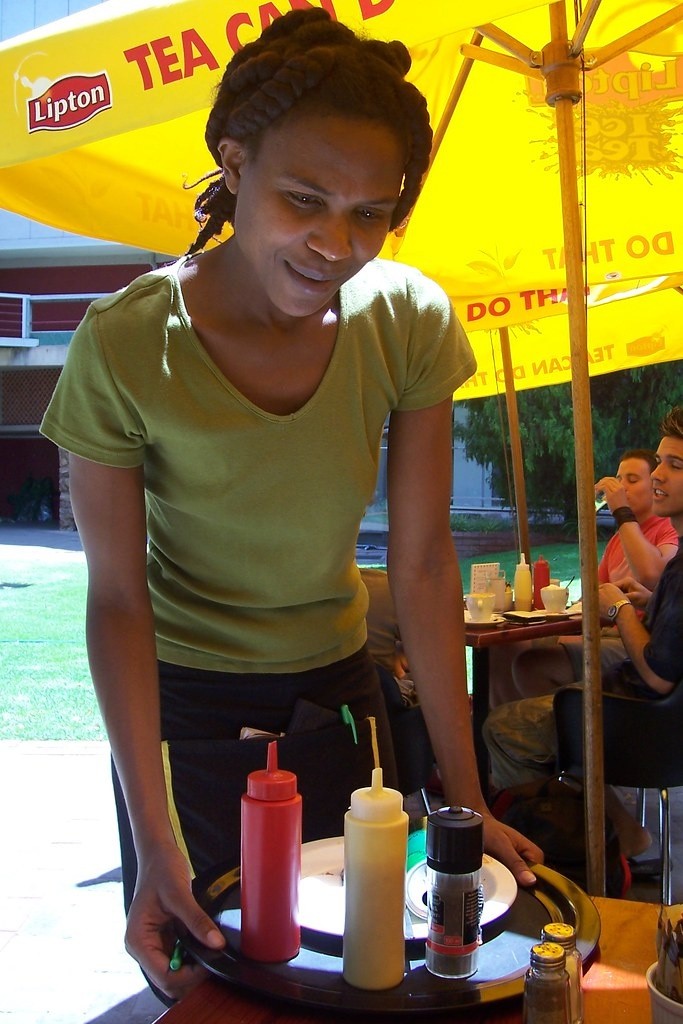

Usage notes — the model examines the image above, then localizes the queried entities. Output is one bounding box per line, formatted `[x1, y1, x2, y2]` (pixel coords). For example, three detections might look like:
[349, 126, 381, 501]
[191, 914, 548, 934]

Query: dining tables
[148, 895, 683, 1024]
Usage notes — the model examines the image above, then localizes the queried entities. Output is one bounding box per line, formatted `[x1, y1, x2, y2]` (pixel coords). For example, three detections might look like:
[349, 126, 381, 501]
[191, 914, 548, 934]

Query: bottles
[533, 555, 550, 610]
[515, 554, 532, 611]
[541, 923, 585, 1024]
[240, 740, 301, 962]
[426, 806, 483, 977]
[344, 767, 409, 990]
[523, 942, 571, 1024]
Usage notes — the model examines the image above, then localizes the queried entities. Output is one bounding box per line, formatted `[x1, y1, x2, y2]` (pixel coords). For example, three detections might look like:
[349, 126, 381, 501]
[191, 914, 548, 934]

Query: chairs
[551, 681, 683, 905]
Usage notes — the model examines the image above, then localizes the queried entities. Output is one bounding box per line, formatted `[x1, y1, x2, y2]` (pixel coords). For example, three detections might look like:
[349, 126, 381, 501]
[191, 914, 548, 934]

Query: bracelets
[613, 506, 639, 527]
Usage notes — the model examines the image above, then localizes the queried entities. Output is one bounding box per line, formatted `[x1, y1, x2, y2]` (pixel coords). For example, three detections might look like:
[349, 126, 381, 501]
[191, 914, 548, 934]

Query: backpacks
[502, 773, 633, 900]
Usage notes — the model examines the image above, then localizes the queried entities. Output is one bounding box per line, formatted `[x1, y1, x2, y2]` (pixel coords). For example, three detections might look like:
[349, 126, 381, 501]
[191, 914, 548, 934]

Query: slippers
[627, 857, 672, 878]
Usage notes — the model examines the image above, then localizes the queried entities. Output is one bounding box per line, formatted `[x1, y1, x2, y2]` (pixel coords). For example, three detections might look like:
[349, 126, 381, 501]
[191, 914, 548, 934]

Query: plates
[464, 617, 504, 628]
[504, 609, 577, 625]
[299, 835, 519, 940]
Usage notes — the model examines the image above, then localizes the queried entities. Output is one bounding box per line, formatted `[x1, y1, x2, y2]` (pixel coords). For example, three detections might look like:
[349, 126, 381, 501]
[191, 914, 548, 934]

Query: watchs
[607, 600, 632, 622]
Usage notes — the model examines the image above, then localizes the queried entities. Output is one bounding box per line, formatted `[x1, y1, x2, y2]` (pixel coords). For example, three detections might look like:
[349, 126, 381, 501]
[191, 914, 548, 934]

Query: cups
[550, 579, 560, 587]
[505, 590, 513, 611]
[465, 594, 495, 622]
[485, 570, 506, 616]
[646, 962, 683, 1024]
[594, 488, 607, 511]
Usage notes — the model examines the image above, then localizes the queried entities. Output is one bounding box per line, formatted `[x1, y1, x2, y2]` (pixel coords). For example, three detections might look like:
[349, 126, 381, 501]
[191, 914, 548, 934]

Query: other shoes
[423, 768, 444, 797]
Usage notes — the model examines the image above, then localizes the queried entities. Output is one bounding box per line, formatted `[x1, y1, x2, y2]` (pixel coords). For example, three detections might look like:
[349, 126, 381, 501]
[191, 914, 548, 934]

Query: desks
[463, 614, 616, 810]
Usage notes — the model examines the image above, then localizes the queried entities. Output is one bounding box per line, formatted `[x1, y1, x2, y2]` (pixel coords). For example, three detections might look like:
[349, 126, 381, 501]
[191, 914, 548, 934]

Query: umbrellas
[449, 271, 683, 575]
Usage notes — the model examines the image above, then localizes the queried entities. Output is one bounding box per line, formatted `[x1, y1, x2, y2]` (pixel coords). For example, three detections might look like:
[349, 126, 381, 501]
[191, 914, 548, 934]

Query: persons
[0, 0, 683, 896]
[489, 448, 679, 710]
[357, 563, 448, 799]
[40, 8, 543, 1008]
[479, 405, 683, 853]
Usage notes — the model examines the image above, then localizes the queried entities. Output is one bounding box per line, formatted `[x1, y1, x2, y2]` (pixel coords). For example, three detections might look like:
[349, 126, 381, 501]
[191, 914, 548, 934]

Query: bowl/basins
[540, 586, 569, 612]
